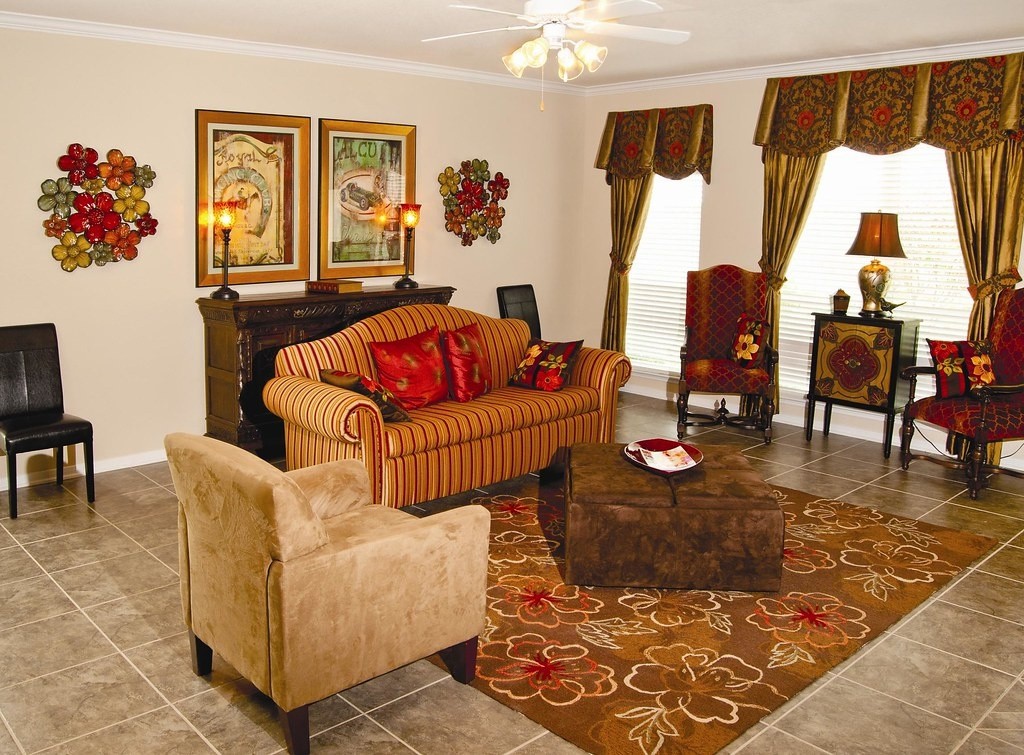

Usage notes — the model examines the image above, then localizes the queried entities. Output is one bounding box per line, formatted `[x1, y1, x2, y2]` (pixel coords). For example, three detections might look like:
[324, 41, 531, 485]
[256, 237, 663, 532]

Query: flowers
[37, 143, 159, 273]
[438, 157, 511, 246]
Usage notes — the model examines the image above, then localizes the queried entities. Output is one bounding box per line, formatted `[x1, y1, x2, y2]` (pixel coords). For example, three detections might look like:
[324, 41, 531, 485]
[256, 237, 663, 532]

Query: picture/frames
[195, 109, 311, 288]
[318, 117, 417, 281]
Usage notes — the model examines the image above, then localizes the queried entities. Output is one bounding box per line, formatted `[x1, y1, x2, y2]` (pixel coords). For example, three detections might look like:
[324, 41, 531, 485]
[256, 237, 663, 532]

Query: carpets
[408, 479, 1001, 755]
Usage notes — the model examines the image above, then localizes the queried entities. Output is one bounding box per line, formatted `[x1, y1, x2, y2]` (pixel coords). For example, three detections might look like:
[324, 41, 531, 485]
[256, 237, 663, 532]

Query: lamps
[846, 209, 909, 320]
[209, 201, 241, 299]
[393, 203, 421, 290]
[501, 35, 608, 113]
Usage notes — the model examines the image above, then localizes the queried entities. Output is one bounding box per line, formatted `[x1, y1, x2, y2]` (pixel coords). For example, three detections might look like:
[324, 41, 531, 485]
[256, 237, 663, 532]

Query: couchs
[163, 431, 490, 755]
[262, 303, 633, 510]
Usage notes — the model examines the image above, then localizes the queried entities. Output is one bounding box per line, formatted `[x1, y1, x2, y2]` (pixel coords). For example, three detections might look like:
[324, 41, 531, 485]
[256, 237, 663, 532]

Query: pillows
[441, 323, 492, 404]
[507, 336, 586, 394]
[925, 337, 1003, 400]
[317, 368, 414, 422]
[727, 313, 772, 372]
[370, 325, 449, 413]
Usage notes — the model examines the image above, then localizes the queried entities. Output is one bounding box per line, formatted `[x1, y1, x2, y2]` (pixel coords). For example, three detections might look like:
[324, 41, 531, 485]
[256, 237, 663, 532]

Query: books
[305, 279, 364, 296]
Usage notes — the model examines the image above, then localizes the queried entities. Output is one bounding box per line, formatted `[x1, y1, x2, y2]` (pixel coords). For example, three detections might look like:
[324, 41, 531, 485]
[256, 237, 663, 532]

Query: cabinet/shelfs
[195, 284, 457, 464]
[805, 312, 924, 460]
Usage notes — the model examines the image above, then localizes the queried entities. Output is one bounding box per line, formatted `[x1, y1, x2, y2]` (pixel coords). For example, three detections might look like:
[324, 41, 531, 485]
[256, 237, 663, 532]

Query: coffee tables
[564, 442, 785, 592]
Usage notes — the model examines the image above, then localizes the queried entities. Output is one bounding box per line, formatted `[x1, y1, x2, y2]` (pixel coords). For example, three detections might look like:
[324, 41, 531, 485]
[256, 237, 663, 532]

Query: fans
[419, 0, 691, 46]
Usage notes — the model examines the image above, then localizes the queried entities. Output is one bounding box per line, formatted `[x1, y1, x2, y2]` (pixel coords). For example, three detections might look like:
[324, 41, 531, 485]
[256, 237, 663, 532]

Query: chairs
[901, 287, 1024, 501]
[674, 264, 780, 445]
[497, 284, 541, 341]
[0, 323, 95, 519]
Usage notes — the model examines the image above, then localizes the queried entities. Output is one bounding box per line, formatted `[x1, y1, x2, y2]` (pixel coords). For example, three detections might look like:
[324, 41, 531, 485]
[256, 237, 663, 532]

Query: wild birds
[880, 298, 907, 318]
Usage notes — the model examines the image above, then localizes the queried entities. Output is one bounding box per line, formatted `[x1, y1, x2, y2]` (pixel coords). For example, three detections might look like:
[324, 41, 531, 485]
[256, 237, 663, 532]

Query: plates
[624, 438, 704, 479]
